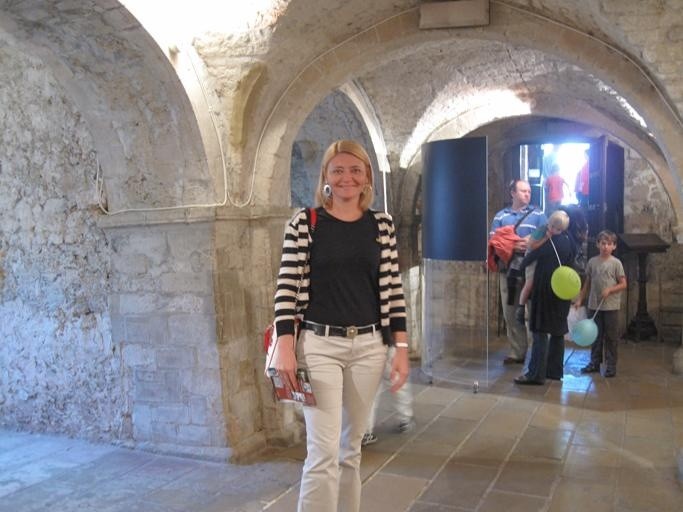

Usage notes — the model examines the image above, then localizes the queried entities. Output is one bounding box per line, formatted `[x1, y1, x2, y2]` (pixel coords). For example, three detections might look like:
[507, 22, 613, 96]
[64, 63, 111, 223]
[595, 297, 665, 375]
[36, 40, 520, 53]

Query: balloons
[571, 319, 599, 348]
[550, 265, 582, 300]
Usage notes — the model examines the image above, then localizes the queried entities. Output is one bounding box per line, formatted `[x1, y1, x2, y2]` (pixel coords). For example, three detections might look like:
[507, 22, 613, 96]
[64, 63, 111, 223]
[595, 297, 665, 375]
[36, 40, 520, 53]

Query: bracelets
[394, 342, 409, 348]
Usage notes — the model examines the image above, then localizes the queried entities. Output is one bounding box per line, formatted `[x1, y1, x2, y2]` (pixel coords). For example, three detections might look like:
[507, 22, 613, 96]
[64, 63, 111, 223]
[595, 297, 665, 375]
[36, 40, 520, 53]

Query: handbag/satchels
[263, 207, 316, 377]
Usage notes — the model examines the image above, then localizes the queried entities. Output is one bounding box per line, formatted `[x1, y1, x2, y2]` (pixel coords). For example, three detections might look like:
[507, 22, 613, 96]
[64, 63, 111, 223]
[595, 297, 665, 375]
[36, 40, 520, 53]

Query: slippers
[514, 376, 544, 387]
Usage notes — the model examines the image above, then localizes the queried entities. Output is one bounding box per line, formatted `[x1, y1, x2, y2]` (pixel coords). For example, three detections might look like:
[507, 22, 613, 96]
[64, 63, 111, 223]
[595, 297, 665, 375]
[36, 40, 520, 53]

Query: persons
[515, 210, 570, 323]
[362, 346, 410, 445]
[266, 137, 409, 512]
[514, 206, 587, 385]
[544, 164, 569, 217]
[574, 230, 627, 377]
[490, 179, 547, 365]
[575, 150, 591, 208]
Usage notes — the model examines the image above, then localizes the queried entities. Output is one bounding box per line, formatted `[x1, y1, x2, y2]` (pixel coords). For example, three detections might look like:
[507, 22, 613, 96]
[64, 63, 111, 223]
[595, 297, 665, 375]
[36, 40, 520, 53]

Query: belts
[299, 320, 382, 338]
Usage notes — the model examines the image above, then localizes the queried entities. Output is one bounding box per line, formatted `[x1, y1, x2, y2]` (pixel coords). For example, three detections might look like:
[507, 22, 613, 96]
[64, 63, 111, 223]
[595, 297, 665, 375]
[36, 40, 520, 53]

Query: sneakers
[578, 361, 599, 374]
[504, 353, 522, 365]
[400, 419, 418, 432]
[603, 369, 617, 377]
[546, 373, 562, 382]
[362, 432, 379, 445]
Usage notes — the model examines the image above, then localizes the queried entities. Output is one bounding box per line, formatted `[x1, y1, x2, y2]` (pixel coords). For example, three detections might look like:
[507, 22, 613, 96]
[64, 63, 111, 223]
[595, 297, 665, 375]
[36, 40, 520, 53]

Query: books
[269, 368, 317, 407]
